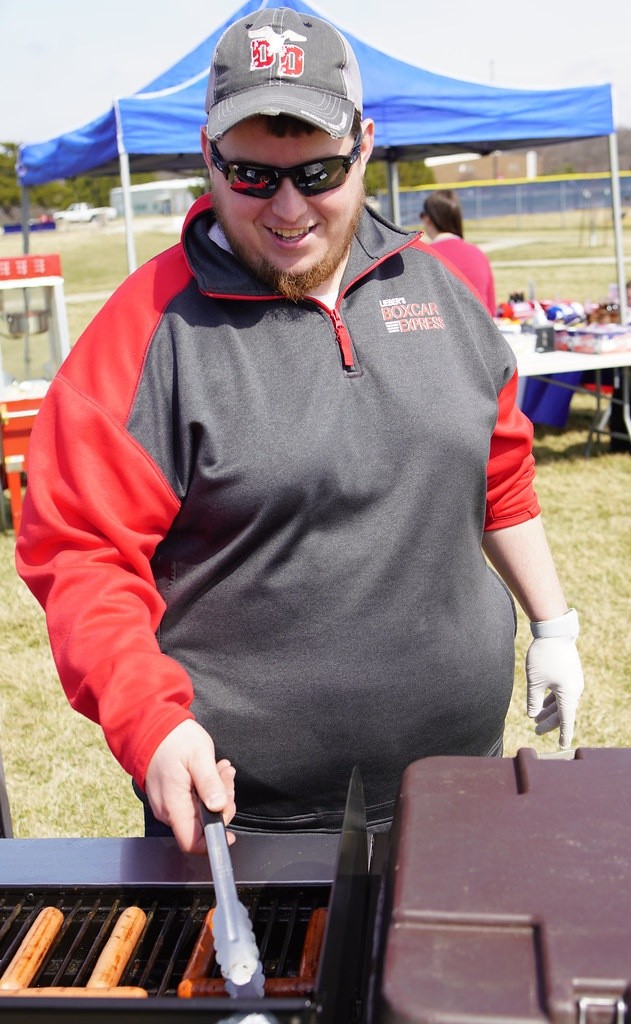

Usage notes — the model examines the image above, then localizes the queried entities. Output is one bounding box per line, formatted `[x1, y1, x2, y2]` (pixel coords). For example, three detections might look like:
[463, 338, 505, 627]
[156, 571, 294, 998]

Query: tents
[17, 2, 613, 184]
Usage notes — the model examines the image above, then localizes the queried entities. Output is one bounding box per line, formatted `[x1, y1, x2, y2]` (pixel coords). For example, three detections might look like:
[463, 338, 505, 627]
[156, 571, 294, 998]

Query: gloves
[522, 606, 587, 752]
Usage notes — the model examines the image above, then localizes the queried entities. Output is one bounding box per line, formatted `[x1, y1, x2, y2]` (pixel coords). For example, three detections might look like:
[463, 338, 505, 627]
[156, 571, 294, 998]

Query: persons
[419, 188, 497, 319]
[14, 7, 587, 857]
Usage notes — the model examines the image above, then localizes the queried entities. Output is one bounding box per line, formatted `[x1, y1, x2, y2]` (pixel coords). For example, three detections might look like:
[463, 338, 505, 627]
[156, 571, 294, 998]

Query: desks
[497, 332, 631, 459]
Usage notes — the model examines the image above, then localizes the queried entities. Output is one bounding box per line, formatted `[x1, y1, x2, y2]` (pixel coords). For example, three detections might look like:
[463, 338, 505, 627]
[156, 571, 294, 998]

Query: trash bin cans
[368, 741, 629, 1024]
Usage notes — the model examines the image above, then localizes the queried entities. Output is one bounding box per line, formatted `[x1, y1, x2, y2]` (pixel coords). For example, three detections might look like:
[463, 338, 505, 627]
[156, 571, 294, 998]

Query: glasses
[210, 135, 362, 197]
[418, 208, 429, 219]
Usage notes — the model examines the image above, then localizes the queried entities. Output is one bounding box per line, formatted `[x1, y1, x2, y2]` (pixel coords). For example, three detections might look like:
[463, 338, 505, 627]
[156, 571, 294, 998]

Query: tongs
[195, 789, 265, 1002]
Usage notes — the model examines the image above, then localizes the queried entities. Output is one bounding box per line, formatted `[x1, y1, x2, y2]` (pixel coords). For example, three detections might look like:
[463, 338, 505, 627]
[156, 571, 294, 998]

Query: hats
[203, 4, 366, 142]
